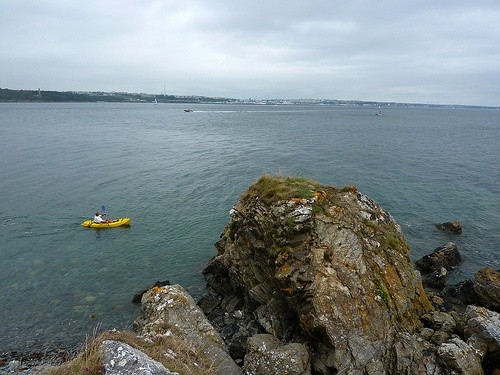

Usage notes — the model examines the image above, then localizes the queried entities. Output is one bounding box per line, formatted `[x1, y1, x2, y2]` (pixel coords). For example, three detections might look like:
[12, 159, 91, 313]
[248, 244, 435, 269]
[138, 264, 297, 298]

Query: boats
[184, 108, 193, 112]
[80, 217, 131, 228]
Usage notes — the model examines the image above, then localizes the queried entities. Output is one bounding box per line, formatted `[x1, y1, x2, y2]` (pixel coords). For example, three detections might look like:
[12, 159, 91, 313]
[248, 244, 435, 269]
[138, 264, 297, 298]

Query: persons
[94, 212, 106, 223]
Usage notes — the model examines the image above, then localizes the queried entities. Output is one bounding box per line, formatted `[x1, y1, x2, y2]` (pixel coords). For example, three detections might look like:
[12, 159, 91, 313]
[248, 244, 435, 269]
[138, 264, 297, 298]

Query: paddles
[101, 205, 110, 229]
